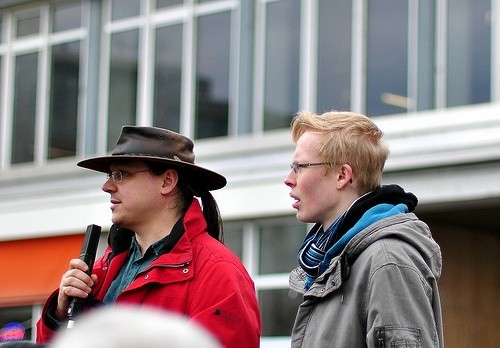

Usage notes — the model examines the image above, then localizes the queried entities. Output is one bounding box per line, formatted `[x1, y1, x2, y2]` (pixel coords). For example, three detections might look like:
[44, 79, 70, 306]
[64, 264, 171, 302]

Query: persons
[30, 122, 263, 348]
[276, 110, 447, 348]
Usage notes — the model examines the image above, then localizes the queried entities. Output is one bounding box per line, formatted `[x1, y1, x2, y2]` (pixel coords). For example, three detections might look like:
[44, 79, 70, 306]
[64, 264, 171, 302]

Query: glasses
[287, 160, 348, 174]
[103, 167, 161, 182]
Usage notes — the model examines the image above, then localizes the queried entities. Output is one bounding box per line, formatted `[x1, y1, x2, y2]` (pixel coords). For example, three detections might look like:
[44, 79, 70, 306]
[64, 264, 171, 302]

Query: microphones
[66, 224, 102, 329]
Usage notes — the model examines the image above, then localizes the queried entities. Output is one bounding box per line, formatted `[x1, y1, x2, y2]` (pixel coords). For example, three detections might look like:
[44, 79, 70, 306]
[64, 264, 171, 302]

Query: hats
[77, 126, 228, 193]
